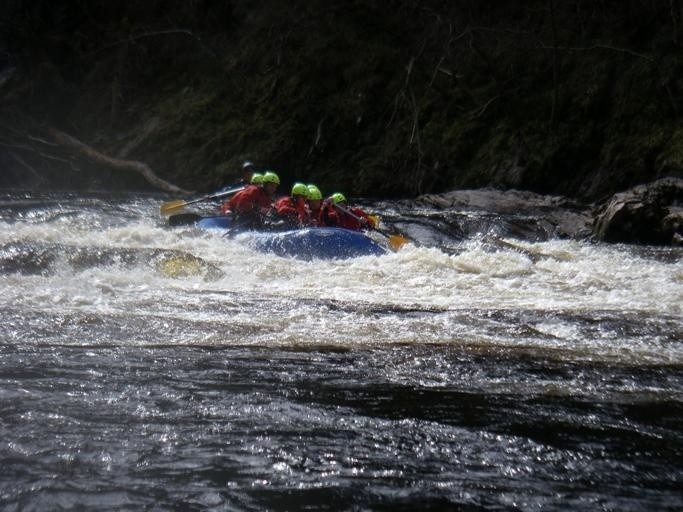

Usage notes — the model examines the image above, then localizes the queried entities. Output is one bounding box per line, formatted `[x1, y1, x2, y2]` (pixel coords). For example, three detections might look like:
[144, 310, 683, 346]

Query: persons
[213, 162, 375, 233]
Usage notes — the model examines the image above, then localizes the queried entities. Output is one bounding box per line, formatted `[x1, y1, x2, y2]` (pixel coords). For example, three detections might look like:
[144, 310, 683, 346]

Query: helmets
[291, 183, 347, 205]
[242, 163, 255, 171]
[262, 173, 280, 185]
[250, 174, 263, 184]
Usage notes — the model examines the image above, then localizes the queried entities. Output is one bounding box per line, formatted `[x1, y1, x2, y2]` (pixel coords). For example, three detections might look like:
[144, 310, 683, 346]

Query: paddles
[333, 203, 410, 252]
[160, 187, 246, 215]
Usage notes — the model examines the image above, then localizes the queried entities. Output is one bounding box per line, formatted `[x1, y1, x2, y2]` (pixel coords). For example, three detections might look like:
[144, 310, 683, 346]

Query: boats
[167, 209, 223, 226]
[188, 215, 391, 265]
